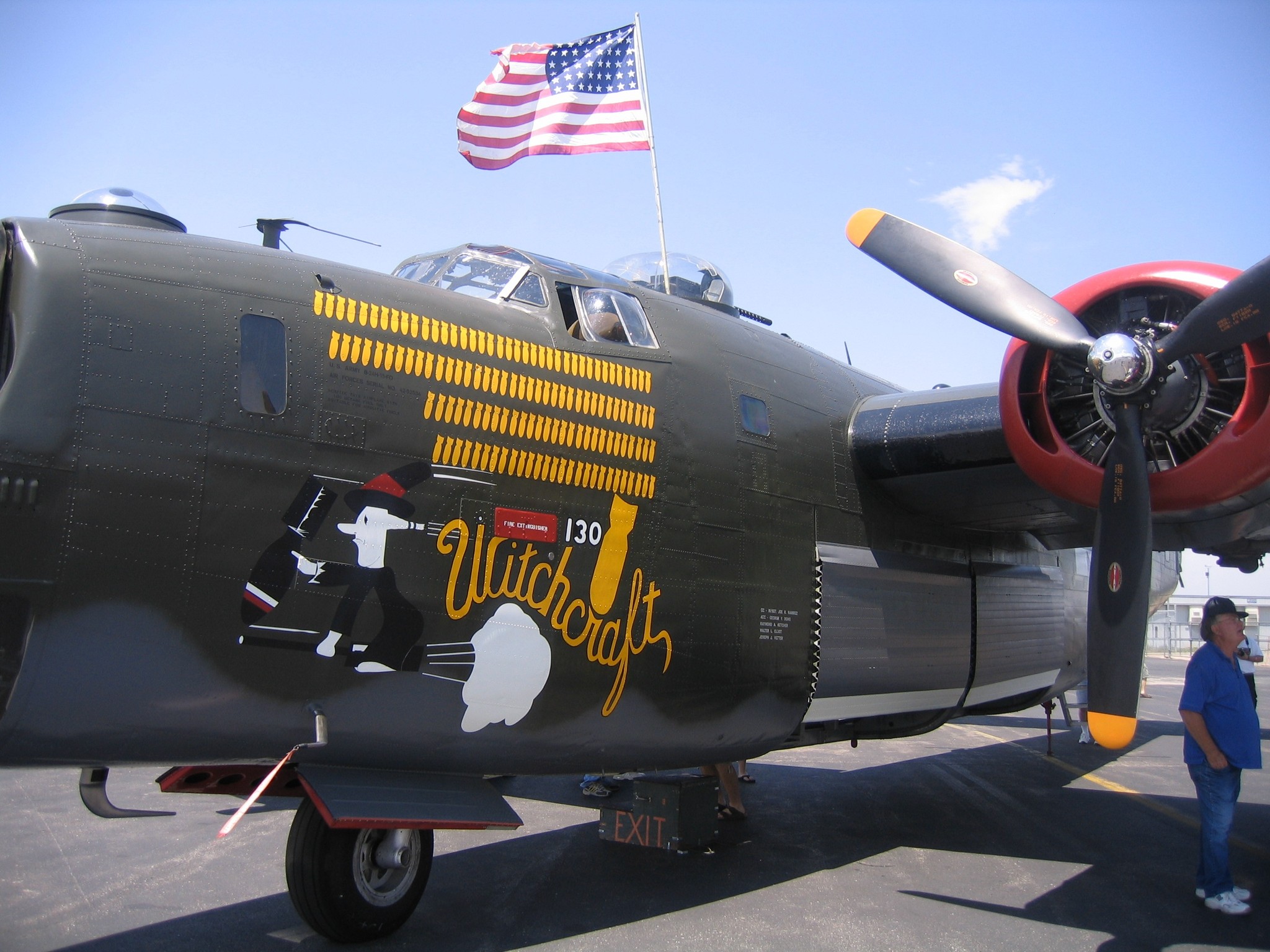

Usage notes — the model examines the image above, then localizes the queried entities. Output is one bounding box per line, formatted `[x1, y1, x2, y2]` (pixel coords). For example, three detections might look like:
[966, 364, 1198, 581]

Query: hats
[1203, 596, 1249, 618]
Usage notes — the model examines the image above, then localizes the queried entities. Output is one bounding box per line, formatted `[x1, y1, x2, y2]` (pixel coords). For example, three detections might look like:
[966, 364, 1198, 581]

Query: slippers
[739, 775, 755, 783]
[717, 806, 748, 823]
[1140, 694, 1152, 698]
[718, 804, 728, 812]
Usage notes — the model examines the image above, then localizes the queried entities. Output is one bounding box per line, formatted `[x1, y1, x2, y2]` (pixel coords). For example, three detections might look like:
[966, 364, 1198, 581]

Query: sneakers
[623, 772, 647, 781]
[1078, 733, 1090, 744]
[1204, 891, 1250, 915]
[1195, 884, 1251, 901]
[613, 772, 629, 780]
[583, 781, 611, 797]
[1094, 741, 1099, 745]
[597, 781, 620, 791]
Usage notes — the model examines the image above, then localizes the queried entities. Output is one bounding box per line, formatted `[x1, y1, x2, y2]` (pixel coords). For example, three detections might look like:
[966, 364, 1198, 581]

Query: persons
[580, 759, 756, 820]
[1076, 677, 1101, 745]
[1180, 597, 1263, 913]
[569, 313, 622, 339]
[1140, 663, 1152, 698]
[1234, 618, 1266, 710]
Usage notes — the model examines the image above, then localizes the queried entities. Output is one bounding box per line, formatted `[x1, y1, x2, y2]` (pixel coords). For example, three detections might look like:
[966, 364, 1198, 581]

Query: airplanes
[2, 175, 1270, 952]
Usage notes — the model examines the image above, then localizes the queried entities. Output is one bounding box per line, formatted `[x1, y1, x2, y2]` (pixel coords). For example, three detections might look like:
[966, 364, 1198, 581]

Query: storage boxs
[600, 775, 719, 852]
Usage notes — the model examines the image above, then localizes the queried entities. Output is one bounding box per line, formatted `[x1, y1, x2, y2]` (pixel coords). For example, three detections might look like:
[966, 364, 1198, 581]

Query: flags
[457, 23, 650, 171]
